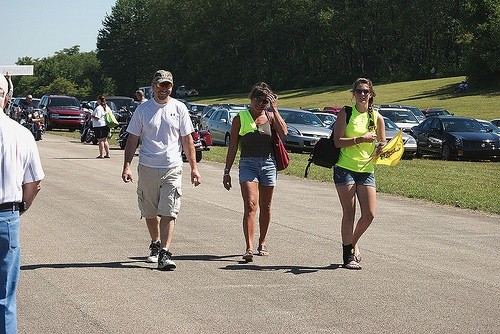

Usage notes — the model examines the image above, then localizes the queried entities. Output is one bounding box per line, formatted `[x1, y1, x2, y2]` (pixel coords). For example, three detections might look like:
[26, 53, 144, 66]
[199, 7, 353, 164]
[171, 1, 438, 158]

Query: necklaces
[356, 113, 367, 121]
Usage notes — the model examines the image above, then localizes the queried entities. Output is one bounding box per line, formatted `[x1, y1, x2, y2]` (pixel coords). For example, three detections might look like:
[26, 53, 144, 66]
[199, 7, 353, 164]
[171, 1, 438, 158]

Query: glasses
[251, 94, 269, 104]
[354, 89, 370, 95]
[153, 82, 173, 89]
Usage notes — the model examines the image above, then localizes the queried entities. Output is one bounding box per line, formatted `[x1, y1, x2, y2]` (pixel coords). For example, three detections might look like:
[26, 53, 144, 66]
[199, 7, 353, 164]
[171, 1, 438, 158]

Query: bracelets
[224, 169, 230, 175]
[353, 136, 357, 146]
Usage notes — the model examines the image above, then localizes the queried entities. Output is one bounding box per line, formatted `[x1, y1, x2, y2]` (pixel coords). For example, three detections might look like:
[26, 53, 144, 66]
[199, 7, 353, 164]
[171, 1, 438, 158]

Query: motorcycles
[19, 104, 45, 141]
[183, 106, 212, 163]
[118, 107, 139, 150]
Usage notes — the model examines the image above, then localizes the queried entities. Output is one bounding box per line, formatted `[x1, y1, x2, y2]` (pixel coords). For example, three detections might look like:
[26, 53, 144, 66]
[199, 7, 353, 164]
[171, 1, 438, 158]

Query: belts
[0, 202, 19, 212]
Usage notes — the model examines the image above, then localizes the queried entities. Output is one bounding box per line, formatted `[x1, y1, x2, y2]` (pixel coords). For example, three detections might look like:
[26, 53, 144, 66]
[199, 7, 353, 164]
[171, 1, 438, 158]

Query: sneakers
[158, 249, 176, 271]
[147, 241, 161, 262]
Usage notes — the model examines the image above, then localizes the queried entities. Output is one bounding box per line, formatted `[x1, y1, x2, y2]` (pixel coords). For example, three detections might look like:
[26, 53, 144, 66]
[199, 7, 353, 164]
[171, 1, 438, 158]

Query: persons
[129, 90, 148, 115]
[452, 81, 470, 94]
[222, 82, 287, 261]
[20, 94, 43, 139]
[89, 96, 111, 158]
[3, 71, 13, 117]
[333, 77, 386, 269]
[0, 73, 45, 334]
[122, 70, 201, 270]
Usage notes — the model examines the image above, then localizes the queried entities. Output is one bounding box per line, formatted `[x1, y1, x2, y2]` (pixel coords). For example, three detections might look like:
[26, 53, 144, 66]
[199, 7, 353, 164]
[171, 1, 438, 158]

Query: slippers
[352, 246, 361, 263]
[342, 260, 362, 270]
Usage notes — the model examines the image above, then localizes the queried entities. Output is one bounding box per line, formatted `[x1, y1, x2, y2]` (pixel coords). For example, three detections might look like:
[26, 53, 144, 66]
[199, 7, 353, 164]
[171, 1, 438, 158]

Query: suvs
[38, 94, 87, 132]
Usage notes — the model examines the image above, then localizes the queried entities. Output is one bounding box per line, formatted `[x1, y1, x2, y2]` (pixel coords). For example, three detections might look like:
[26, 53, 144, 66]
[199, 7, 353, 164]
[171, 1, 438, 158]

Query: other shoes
[242, 250, 253, 262]
[257, 244, 269, 256]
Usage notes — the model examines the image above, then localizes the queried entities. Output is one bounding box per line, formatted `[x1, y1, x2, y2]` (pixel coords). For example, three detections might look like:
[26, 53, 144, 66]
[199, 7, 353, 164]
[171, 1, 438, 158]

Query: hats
[152, 70, 174, 86]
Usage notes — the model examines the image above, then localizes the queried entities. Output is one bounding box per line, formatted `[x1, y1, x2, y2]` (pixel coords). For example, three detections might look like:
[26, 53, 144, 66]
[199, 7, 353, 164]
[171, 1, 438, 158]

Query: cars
[266, 107, 333, 153]
[420, 108, 455, 118]
[380, 104, 425, 123]
[79, 96, 141, 124]
[300, 106, 343, 127]
[373, 107, 421, 136]
[178, 99, 251, 132]
[176, 85, 199, 98]
[475, 118, 500, 137]
[5, 97, 42, 122]
[409, 116, 500, 161]
[205, 109, 247, 148]
[383, 117, 417, 160]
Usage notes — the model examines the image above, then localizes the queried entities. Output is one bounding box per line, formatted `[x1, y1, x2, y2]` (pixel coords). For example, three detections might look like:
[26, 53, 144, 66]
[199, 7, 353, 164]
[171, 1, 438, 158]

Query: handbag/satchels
[105, 111, 119, 128]
[303, 105, 352, 178]
[265, 110, 290, 171]
